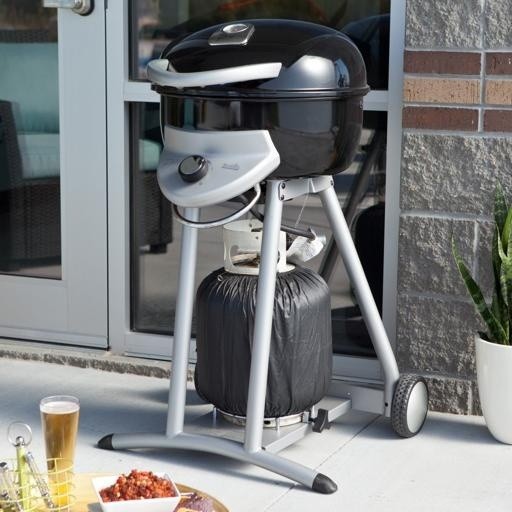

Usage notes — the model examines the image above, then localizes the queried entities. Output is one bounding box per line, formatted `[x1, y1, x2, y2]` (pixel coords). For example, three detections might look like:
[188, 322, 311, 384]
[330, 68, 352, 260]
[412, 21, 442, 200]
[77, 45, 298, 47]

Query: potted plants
[451, 181, 512, 445]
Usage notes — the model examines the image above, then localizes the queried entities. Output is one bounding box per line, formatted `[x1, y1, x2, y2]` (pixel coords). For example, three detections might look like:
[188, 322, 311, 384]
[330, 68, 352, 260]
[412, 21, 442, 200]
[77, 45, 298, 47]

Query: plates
[91, 472, 180, 512]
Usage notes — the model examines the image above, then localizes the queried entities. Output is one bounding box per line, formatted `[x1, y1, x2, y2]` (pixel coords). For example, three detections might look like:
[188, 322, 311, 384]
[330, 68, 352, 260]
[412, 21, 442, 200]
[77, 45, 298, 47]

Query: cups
[39, 395, 79, 473]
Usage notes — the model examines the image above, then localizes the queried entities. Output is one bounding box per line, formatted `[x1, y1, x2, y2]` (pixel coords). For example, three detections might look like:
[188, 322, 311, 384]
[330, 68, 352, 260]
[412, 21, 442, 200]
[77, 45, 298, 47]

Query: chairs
[0, 41, 176, 272]
